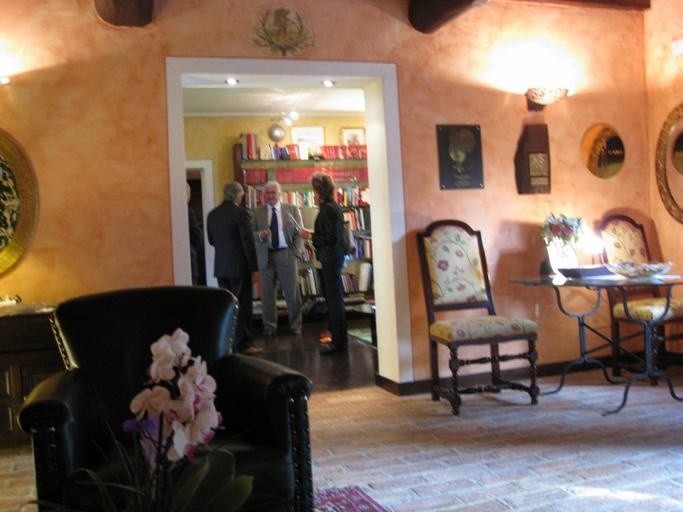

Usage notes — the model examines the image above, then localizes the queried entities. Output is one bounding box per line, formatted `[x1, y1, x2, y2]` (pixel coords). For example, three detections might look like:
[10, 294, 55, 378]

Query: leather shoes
[263, 330, 273, 338]
[246, 347, 263, 353]
[291, 328, 301, 334]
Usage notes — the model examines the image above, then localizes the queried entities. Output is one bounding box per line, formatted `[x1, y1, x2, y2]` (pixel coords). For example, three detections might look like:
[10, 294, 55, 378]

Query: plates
[559, 265, 615, 277]
[615, 262, 672, 278]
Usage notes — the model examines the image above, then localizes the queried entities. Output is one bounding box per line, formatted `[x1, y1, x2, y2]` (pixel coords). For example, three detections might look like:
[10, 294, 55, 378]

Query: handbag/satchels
[343, 221, 355, 254]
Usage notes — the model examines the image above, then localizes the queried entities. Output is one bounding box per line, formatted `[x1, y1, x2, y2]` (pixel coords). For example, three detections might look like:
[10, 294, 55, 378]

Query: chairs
[416, 219, 540, 417]
[594, 214, 683, 387]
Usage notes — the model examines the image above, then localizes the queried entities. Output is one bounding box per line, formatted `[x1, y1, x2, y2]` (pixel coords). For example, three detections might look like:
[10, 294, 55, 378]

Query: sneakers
[320, 344, 346, 356]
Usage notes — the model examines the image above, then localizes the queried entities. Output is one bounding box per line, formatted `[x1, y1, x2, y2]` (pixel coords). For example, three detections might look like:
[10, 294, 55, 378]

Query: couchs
[17, 286, 314, 512]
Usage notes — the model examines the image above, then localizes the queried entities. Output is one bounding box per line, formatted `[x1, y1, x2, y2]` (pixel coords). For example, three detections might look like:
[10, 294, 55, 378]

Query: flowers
[73, 329, 254, 512]
[538, 211, 583, 246]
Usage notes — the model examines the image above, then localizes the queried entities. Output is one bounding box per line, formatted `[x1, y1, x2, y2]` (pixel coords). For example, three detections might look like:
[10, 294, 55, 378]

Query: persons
[251, 181, 306, 336]
[299, 173, 349, 356]
[207, 182, 264, 354]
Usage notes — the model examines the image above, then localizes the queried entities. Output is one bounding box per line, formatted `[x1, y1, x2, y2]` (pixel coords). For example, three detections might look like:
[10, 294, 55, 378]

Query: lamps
[526, 88, 569, 106]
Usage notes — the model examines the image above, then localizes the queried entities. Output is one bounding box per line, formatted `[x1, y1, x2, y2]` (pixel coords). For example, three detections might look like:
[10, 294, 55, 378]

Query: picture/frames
[436, 123, 485, 190]
[291, 125, 325, 145]
[655, 103, 683, 226]
[340, 127, 367, 145]
[0, 128, 40, 275]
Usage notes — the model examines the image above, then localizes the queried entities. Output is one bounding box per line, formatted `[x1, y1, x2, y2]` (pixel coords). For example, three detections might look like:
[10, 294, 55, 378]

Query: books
[246, 186, 359, 210]
[321, 144, 366, 159]
[300, 268, 359, 296]
[242, 132, 302, 160]
[344, 208, 365, 231]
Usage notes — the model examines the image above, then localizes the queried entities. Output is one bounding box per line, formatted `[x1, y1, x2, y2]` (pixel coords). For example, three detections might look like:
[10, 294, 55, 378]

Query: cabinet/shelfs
[1, 302, 65, 451]
[233, 143, 372, 317]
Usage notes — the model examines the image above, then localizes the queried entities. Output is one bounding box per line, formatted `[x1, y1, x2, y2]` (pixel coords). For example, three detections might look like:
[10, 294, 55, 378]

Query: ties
[270, 208, 279, 248]
